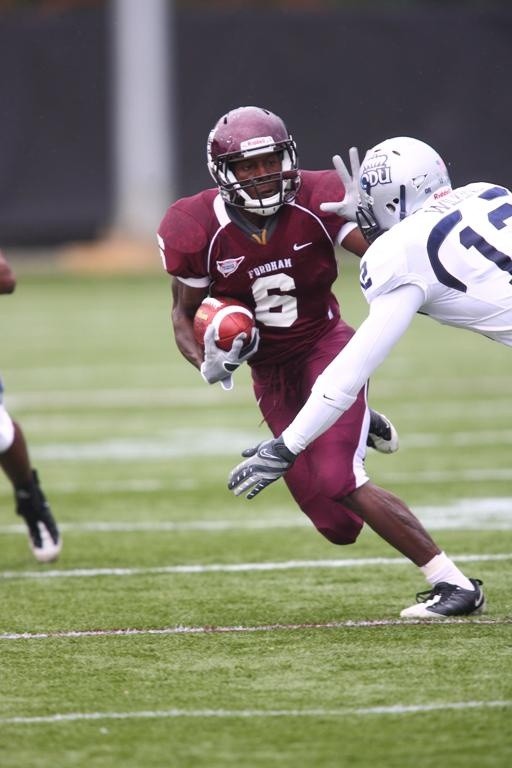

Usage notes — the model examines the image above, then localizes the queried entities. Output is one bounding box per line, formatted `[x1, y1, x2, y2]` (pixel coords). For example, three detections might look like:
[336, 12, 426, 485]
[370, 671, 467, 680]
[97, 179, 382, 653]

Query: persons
[227, 136, 512, 500]
[0, 241, 62, 564]
[155, 105, 487, 617]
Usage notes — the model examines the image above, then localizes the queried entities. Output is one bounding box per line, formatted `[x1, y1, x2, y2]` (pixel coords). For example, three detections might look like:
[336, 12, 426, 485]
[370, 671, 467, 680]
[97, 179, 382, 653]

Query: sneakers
[398, 579, 487, 619]
[366, 409, 400, 454]
[16, 470, 63, 563]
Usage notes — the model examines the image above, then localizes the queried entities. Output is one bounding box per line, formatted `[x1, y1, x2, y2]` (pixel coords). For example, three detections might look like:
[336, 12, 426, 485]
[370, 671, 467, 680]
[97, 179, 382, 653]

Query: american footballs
[193, 296, 256, 351]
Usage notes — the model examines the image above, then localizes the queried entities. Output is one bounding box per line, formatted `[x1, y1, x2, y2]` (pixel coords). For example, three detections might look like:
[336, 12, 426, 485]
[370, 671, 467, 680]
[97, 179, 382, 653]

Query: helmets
[205, 105, 302, 217]
[355, 136, 454, 245]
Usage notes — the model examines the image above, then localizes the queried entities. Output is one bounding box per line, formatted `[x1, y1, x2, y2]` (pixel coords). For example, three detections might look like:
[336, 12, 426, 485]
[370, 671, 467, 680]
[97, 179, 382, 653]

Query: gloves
[197, 324, 260, 393]
[227, 435, 298, 502]
[319, 144, 364, 223]
